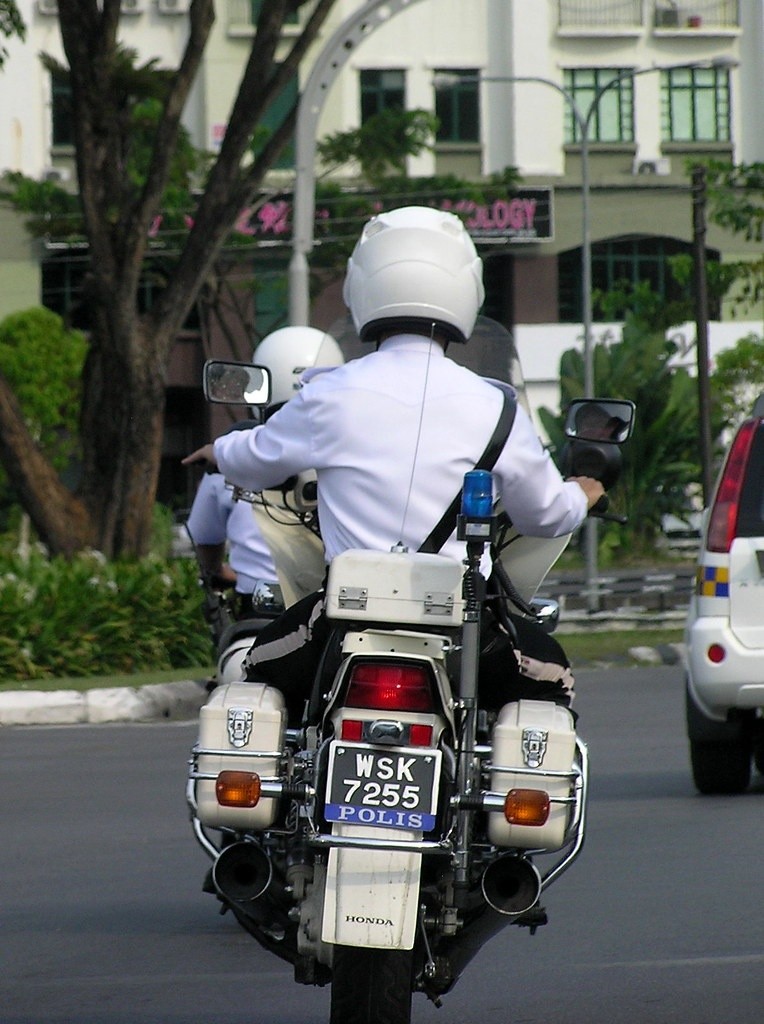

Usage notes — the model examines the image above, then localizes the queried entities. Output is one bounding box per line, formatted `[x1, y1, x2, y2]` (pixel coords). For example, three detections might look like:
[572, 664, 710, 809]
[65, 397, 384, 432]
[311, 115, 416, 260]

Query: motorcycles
[192, 566, 288, 658]
[182, 357, 636, 1024]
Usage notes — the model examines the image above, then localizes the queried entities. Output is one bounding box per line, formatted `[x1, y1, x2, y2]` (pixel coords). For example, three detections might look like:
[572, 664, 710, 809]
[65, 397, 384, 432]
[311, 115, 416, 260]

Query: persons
[190, 327, 346, 596]
[182, 205, 605, 708]
[569, 403, 631, 442]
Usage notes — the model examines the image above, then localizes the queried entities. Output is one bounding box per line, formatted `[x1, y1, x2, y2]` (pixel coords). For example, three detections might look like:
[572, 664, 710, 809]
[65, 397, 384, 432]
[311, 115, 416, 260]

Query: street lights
[431, 51, 743, 615]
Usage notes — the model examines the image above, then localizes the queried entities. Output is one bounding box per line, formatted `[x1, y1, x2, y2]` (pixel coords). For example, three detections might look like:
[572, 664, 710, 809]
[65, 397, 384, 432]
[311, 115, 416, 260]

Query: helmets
[342, 205, 487, 345]
[251, 324, 345, 427]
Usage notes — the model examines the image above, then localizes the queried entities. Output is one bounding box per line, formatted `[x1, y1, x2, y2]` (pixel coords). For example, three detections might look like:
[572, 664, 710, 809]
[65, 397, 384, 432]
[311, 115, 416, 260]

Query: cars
[682, 391, 764, 794]
